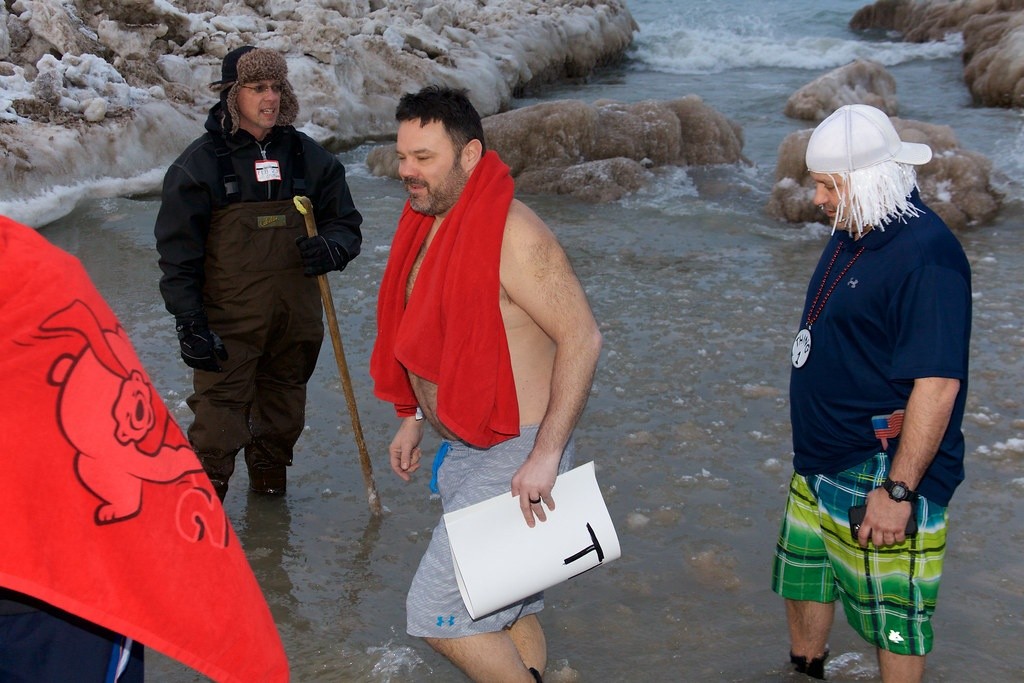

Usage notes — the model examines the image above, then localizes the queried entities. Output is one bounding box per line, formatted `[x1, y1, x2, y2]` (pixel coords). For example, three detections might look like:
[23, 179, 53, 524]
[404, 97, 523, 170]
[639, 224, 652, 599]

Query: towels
[0, 212, 290, 682]
[367, 148, 520, 451]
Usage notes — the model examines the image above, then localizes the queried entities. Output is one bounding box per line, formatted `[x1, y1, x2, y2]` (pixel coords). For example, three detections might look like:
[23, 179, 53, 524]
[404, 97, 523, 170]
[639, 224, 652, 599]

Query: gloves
[296, 234, 347, 274]
[175, 312, 229, 373]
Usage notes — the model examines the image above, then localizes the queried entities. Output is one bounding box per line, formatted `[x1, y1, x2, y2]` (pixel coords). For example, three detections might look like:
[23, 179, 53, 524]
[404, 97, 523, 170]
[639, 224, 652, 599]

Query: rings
[529, 497, 541, 504]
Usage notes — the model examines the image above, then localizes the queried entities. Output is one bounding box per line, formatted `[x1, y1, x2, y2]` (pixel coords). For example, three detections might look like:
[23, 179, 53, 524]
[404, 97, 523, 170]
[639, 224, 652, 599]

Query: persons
[155, 44, 364, 505]
[388, 82, 604, 682]
[771, 104, 974, 683]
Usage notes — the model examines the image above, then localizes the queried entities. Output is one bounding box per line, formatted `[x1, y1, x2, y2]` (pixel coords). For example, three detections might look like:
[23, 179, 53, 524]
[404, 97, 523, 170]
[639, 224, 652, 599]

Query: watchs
[883, 478, 919, 502]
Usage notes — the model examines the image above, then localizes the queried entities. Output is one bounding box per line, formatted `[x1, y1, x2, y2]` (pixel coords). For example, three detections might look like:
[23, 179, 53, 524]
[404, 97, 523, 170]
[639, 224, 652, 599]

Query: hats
[806, 103, 932, 173]
[209, 46, 299, 136]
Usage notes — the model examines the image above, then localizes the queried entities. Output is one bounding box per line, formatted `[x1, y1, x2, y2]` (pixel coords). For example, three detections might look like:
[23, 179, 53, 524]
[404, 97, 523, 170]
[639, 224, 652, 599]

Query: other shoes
[791, 647, 830, 682]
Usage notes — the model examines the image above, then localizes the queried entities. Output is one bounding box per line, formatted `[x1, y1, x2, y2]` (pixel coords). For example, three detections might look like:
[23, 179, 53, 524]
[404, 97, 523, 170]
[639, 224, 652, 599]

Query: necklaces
[790, 240, 866, 368]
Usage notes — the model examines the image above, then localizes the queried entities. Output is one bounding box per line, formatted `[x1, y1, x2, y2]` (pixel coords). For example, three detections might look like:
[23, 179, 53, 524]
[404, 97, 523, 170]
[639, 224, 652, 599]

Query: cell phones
[848, 504, 918, 541]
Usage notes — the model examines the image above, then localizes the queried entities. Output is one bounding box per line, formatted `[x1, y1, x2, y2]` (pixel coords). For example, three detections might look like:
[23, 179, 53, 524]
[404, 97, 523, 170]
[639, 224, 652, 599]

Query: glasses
[241, 84, 283, 92]
